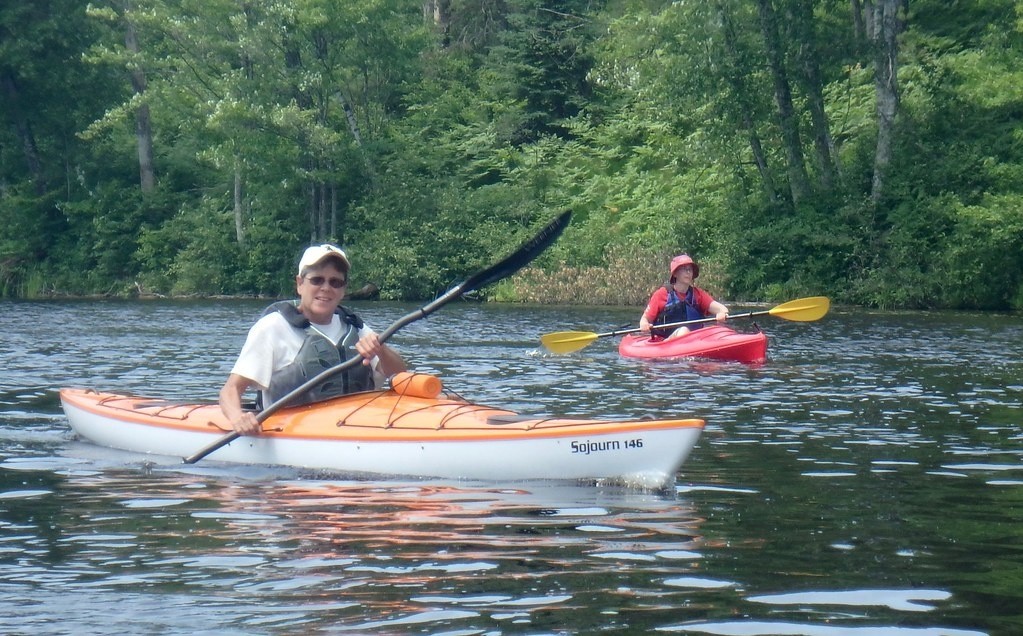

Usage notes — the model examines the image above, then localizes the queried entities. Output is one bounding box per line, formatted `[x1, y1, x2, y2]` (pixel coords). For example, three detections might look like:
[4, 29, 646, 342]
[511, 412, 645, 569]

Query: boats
[619, 325, 768, 364]
[59, 375, 708, 482]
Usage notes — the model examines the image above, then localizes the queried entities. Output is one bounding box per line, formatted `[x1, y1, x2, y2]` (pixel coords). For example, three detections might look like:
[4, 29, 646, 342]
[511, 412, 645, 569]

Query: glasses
[301, 275, 346, 288]
[678, 268, 694, 273]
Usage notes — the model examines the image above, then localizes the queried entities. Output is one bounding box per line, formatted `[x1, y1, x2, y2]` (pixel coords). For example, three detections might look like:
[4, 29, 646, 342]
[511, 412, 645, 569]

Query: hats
[298, 244, 352, 278]
[669, 255, 700, 285]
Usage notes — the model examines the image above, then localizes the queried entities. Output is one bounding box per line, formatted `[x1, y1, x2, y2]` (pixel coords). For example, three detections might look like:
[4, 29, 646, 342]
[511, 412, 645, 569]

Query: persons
[219, 244, 406, 435]
[639, 254, 730, 342]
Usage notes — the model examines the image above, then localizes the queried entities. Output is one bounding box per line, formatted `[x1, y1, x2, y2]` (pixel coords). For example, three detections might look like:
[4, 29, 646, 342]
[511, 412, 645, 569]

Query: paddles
[540, 295, 829, 355]
[183, 209, 573, 464]
